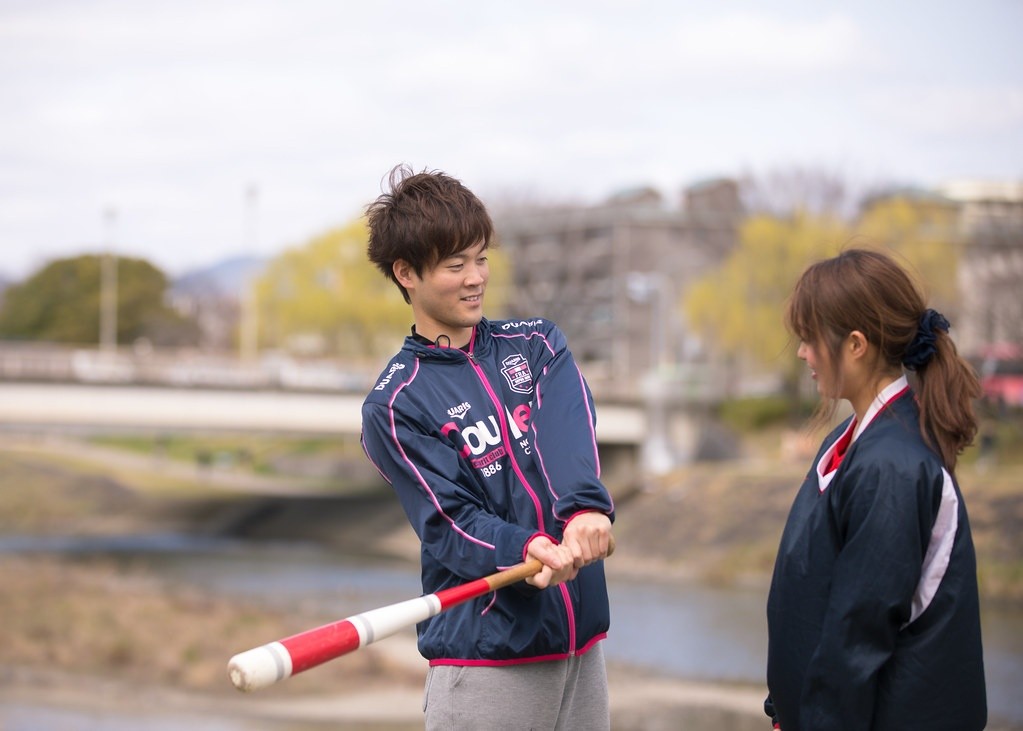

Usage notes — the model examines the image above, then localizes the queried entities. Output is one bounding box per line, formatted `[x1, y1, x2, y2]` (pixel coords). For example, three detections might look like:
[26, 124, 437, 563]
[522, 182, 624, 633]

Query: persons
[360, 163, 617, 730]
[762, 251, 988, 731]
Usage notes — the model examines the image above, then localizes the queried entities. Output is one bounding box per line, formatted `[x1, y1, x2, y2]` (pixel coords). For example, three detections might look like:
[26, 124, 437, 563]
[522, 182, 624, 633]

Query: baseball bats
[224, 533, 616, 697]
